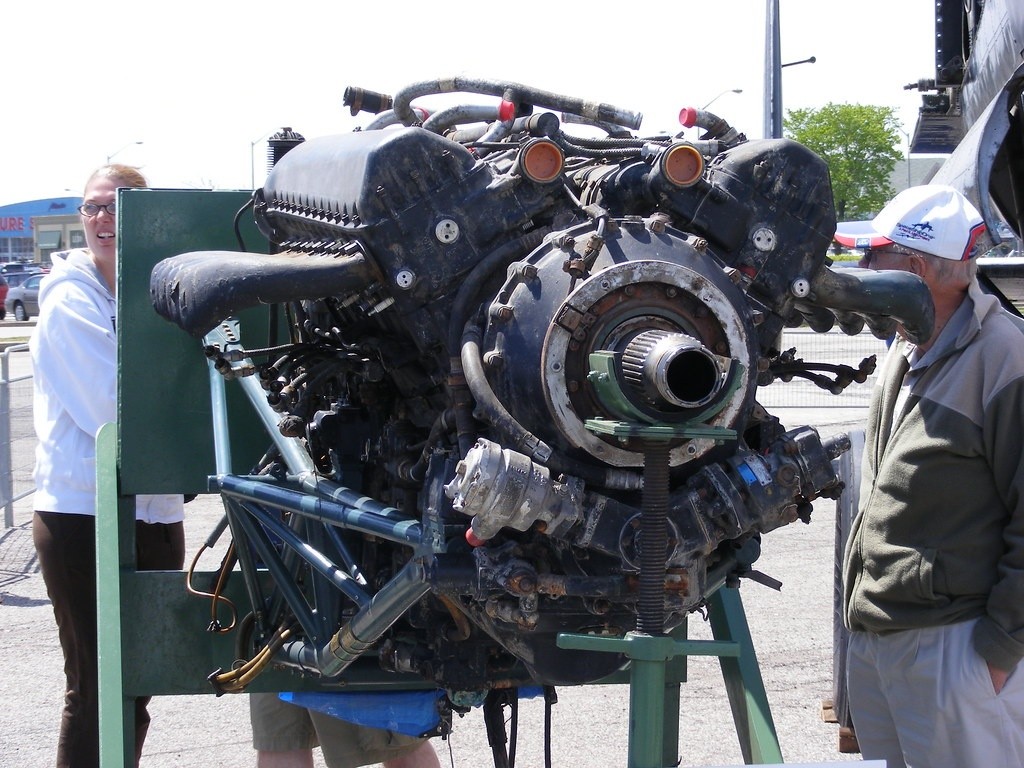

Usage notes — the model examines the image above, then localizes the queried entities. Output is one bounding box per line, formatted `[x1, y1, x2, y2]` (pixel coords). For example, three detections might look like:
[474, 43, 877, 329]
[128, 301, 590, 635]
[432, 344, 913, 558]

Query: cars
[0, 262, 51, 322]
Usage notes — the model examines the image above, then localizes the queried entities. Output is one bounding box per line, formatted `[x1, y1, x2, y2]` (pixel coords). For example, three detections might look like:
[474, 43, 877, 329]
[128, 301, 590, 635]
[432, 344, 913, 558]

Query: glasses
[863, 248, 913, 262]
[77, 200, 119, 217]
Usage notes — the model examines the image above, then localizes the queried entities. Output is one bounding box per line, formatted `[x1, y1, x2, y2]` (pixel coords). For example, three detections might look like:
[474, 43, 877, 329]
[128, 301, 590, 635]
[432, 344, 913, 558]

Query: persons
[834, 184, 1024, 767]
[33, 164, 201, 768]
[249, 688, 441, 768]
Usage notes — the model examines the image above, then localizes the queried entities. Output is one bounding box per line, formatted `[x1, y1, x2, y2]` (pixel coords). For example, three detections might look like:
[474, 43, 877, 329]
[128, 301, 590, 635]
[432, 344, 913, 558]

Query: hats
[832, 183, 988, 261]
